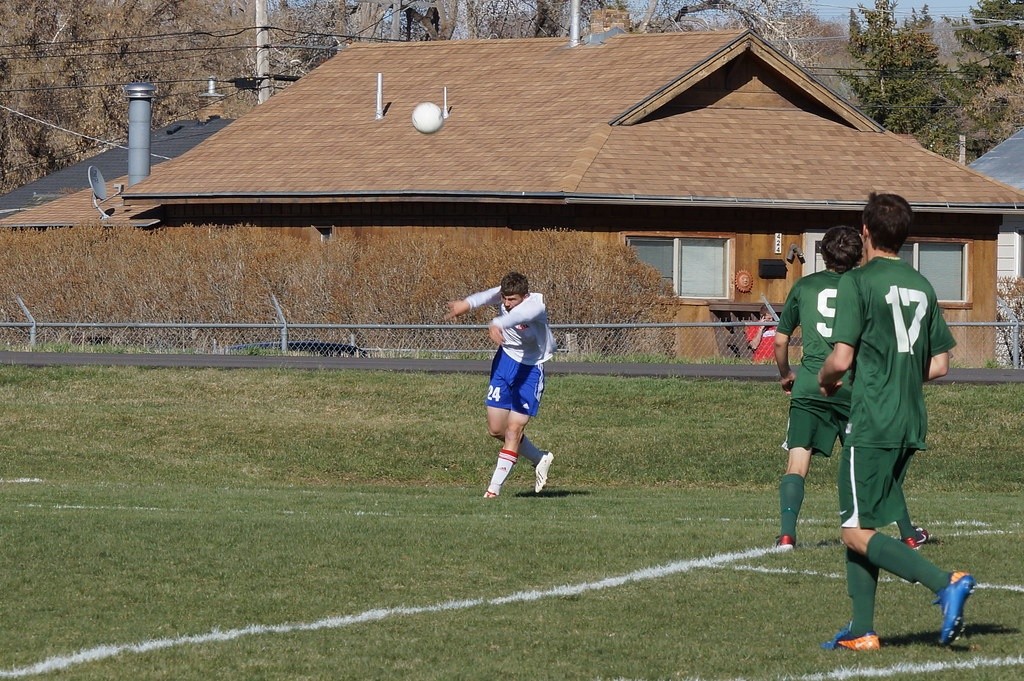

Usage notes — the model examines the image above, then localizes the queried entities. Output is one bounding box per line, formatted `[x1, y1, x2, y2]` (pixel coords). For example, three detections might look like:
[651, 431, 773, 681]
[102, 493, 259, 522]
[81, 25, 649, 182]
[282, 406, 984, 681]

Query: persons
[443, 272, 558, 497]
[775, 226, 929, 550]
[815, 191, 974, 654]
[746, 303, 778, 364]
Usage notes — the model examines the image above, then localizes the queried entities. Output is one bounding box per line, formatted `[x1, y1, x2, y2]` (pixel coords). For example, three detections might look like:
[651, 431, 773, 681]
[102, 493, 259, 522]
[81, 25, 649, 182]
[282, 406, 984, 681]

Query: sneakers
[900, 527, 930, 550]
[535, 451, 554, 493]
[774, 534, 794, 550]
[820, 631, 881, 651]
[932, 572, 975, 645]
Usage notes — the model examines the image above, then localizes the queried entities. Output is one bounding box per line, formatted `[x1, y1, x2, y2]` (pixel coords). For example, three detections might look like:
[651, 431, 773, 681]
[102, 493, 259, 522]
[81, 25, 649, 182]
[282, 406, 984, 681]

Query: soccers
[412, 102, 444, 134]
